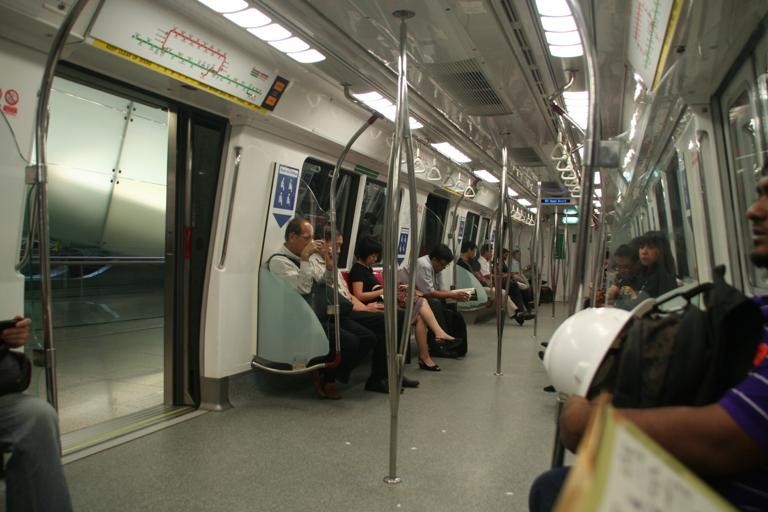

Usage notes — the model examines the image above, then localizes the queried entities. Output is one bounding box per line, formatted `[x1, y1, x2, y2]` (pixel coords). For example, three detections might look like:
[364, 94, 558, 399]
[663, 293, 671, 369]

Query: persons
[1, 316, 75, 510]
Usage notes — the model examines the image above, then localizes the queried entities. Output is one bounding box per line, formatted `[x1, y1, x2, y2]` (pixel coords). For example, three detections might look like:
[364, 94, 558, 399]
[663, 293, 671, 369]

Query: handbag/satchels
[305, 283, 353, 319]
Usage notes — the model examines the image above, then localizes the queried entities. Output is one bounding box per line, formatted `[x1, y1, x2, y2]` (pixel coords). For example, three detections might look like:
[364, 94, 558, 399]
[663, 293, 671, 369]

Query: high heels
[418, 355, 442, 372]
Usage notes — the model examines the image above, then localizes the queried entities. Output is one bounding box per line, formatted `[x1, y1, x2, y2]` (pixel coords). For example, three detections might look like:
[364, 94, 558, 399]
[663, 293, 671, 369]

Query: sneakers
[364, 381, 404, 394]
[311, 373, 342, 400]
[401, 376, 419, 388]
[514, 311, 536, 326]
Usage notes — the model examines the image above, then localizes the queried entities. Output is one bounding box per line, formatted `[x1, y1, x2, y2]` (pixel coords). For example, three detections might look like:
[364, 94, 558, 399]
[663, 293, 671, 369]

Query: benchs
[451, 265, 492, 326]
[256, 261, 384, 377]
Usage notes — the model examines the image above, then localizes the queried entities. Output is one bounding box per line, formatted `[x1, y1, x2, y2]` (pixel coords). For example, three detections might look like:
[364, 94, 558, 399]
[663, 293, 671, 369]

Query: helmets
[542, 296, 659, 402]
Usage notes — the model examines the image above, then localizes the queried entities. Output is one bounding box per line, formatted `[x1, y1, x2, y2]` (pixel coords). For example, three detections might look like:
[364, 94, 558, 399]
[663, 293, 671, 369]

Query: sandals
[433, 333, 461, 346]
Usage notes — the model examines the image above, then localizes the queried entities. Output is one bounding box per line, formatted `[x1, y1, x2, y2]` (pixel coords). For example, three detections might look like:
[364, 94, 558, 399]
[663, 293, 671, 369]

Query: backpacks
[612, 264, 763, 409]
[426, 308, 469, 358]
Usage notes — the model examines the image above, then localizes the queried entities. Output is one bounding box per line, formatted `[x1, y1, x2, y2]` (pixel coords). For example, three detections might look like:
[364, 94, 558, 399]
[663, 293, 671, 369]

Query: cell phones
[0, 319, 20, 349]
